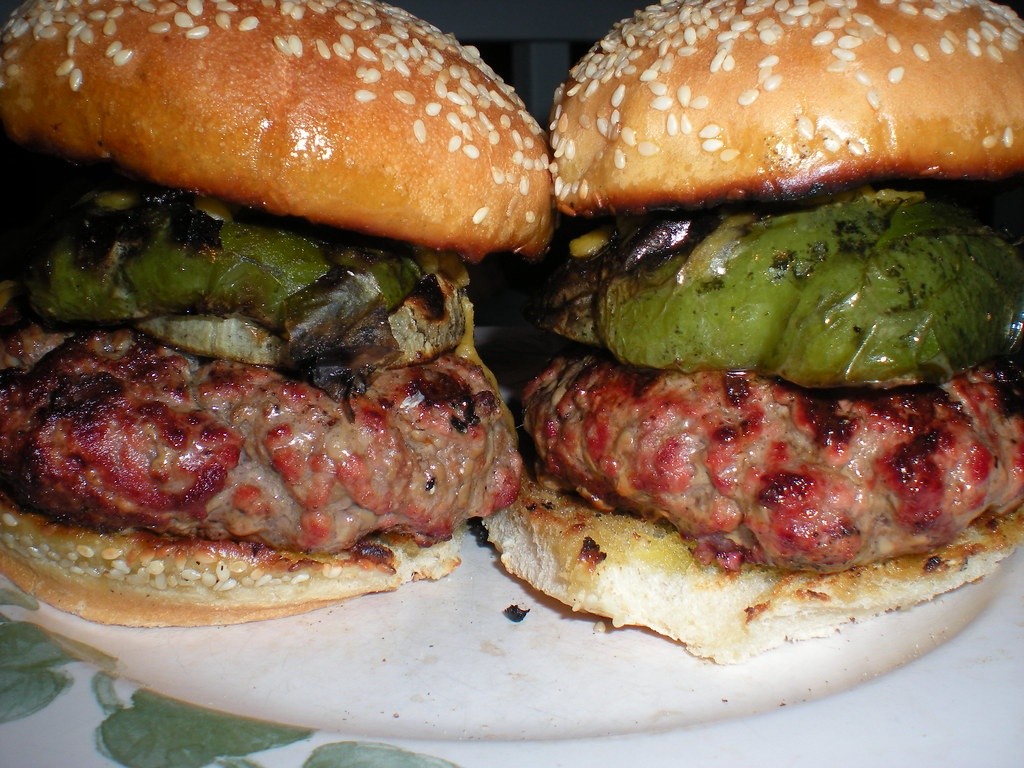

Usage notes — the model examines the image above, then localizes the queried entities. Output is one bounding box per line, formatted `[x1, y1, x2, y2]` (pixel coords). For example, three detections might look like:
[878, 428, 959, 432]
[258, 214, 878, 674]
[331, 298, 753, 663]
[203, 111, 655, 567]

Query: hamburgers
[1, 0, 554, 628]
[481, 0, 1024, 666]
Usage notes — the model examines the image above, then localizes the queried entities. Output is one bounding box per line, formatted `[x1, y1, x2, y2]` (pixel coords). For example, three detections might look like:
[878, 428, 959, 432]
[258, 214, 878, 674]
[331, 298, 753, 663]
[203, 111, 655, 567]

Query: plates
[0, 523, 1011, 742]
